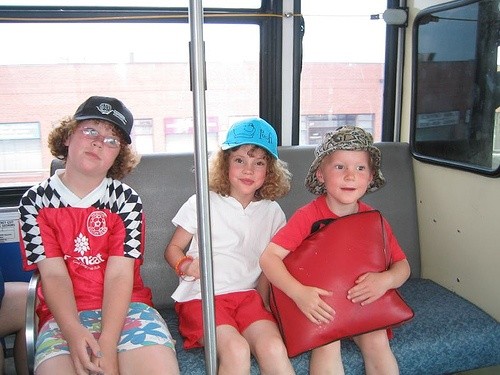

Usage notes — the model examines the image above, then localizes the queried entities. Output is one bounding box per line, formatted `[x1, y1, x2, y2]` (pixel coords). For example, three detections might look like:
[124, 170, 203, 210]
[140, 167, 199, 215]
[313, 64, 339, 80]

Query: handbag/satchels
[268, 210, 416, 359]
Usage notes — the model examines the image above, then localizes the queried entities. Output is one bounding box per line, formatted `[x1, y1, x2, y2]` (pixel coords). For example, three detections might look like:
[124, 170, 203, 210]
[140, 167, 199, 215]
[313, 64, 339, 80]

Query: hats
[72, 96, 133, 144]
[221, 117, 279, 159]
[303, 125, 386, 196]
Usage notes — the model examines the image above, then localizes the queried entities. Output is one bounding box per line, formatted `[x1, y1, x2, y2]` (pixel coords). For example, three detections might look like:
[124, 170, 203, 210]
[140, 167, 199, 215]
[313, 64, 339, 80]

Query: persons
[259, 125, 410, 375]
[0, 282, 39, 375]
[18, 96, 180, 375]
[165, 118, 293, 375]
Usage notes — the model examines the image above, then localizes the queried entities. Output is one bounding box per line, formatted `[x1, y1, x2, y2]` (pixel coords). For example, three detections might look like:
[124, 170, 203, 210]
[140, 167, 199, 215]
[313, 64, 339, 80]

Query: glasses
[81, 126, 120, 148]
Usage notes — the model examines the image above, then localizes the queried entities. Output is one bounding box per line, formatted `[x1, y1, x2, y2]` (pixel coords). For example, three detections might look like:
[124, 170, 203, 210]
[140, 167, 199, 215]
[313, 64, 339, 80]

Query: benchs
[26, 142, 500, 375]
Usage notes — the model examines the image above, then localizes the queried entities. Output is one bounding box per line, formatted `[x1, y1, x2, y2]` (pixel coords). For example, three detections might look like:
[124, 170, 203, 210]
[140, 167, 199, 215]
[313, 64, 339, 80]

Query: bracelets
[175, 256, 197, 282]
